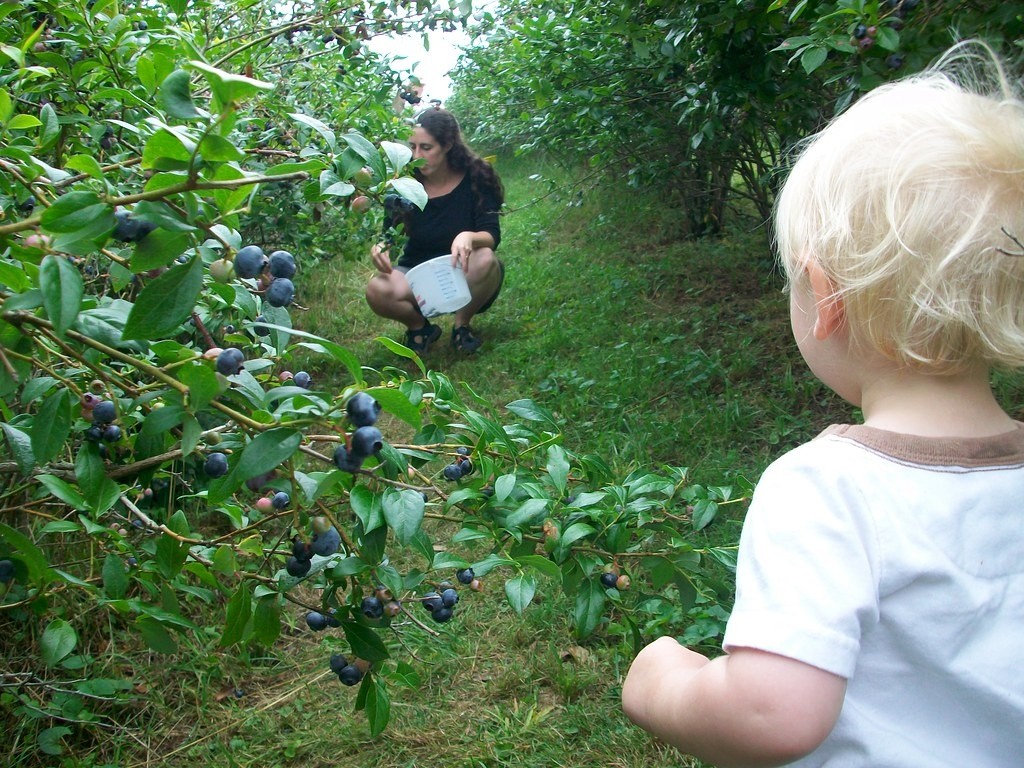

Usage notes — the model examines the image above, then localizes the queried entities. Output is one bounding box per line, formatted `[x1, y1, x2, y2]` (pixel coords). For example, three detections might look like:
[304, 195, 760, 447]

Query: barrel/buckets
[403, 253, 473, 318]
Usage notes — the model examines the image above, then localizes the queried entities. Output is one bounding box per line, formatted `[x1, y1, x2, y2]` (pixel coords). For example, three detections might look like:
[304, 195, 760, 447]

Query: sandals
[396, 320, 442, 363]
[450, 324, 481, 356]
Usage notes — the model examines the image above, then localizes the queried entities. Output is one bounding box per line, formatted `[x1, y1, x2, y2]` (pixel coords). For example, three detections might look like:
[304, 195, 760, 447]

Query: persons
[364, 107, 505, 361]
[619, 40, 1024, 767]
